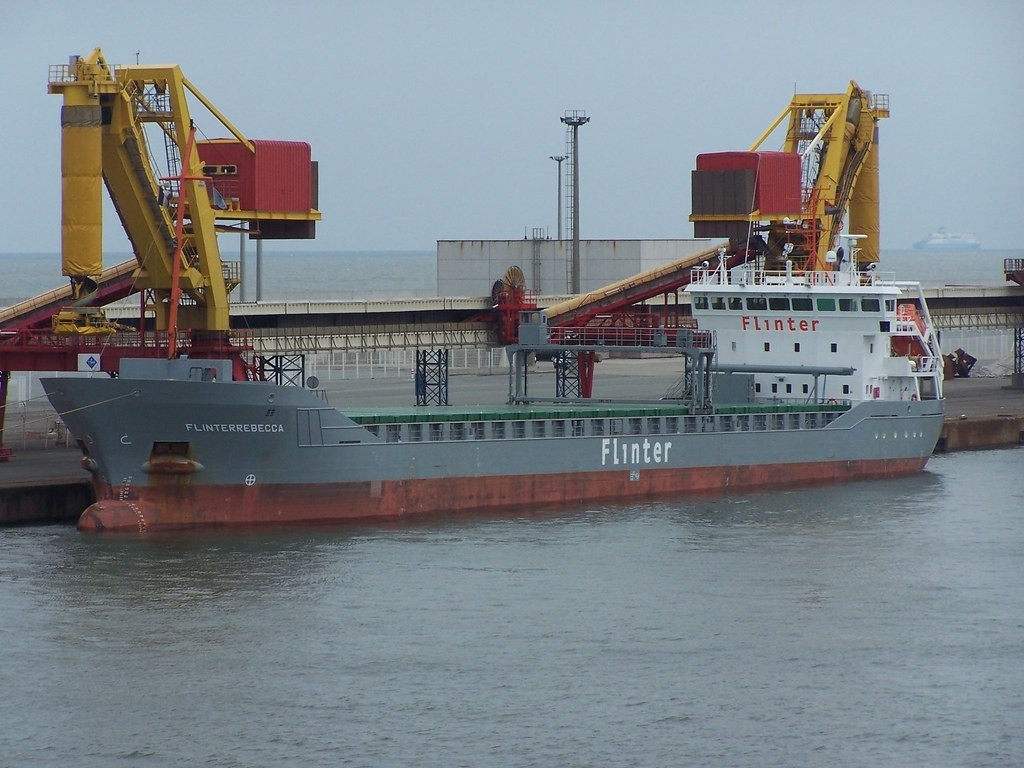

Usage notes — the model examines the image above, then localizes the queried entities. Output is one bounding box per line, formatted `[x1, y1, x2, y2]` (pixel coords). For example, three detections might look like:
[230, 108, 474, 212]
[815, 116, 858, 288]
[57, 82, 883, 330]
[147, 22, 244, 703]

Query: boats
[35, 231, 948, 531]
